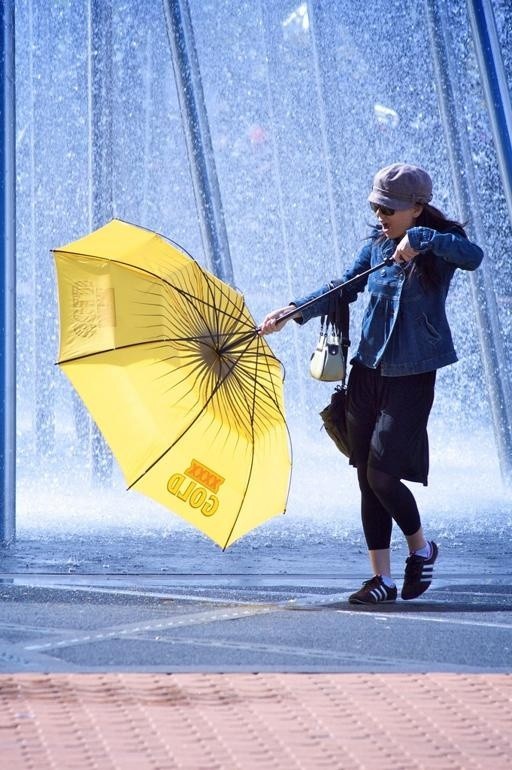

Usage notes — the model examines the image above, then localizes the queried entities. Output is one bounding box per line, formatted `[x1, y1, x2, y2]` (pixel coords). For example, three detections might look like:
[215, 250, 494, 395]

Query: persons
[258, 163, 483, 604]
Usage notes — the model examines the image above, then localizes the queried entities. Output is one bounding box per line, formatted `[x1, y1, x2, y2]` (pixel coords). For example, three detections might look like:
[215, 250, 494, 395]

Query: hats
[367, 164, 433, 211]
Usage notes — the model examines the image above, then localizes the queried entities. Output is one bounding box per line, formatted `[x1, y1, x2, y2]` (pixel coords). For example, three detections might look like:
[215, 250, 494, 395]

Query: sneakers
[349, 575, 398, 605]
[401, 541, 438, 601]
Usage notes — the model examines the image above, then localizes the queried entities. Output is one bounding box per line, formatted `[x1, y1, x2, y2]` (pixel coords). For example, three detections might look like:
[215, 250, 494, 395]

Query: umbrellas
[49, 218, 393, 553]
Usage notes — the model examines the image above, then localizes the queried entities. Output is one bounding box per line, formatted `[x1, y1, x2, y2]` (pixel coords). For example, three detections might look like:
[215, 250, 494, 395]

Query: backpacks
[319, 389, 429, 487]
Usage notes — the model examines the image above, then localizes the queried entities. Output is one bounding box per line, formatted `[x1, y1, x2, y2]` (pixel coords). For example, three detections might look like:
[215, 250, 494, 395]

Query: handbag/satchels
[309, 282, 347, 382]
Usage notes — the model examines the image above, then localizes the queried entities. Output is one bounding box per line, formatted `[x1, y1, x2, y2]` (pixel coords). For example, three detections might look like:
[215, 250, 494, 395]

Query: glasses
[370, 201, 395, 217]
